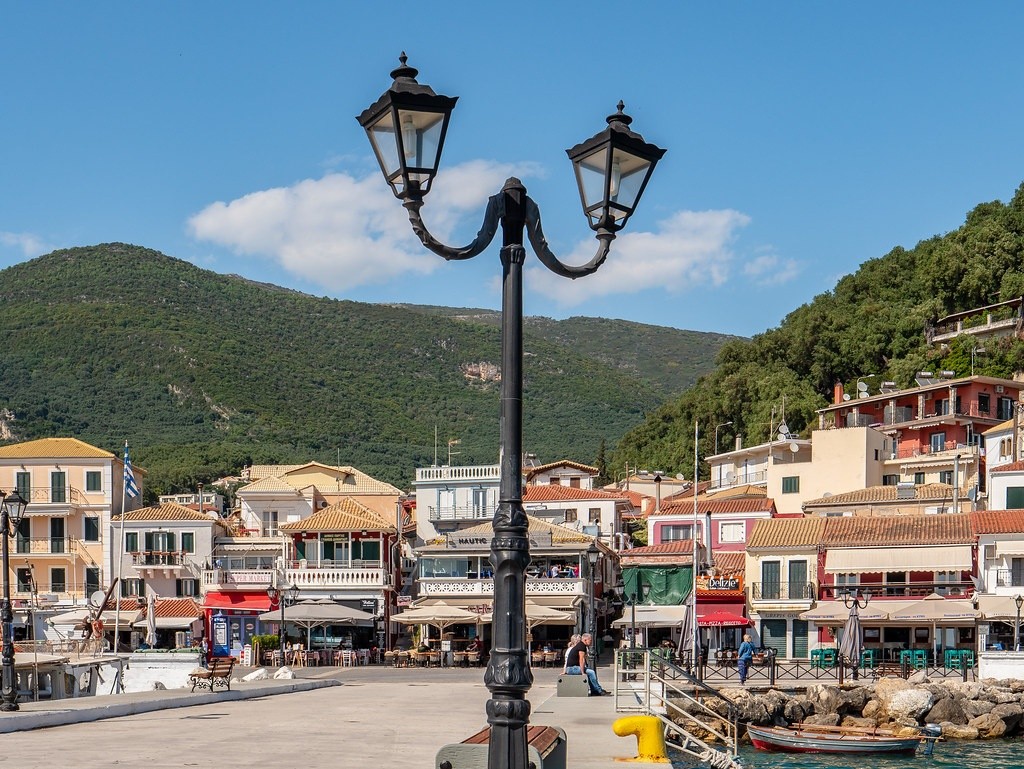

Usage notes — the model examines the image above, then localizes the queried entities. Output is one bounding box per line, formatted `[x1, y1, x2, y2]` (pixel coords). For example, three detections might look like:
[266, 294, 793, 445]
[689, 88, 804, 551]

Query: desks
[283, 650, 291, 666]
[337, 652, 357, 667]
[301, 652, 320, 667]
[433, 571, 570, 578]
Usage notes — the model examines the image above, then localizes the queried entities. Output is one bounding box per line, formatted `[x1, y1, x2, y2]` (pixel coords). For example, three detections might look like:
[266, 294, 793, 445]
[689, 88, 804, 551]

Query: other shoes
[601, 691, 611, 696]
[591, 691, 601, 696]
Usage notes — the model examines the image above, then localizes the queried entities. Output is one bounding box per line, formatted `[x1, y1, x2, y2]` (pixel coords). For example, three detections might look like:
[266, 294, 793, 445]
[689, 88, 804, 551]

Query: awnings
[696, 604, 743, 618]
[825, 546, 973, 574]
[996, 540, 1024, 559]
[815, 602, 975, 622]
[199, 594, 272, 611]
[977, 597, 1024, 622]
[615, 608, 686, 629]
[751, 603, 811, 610]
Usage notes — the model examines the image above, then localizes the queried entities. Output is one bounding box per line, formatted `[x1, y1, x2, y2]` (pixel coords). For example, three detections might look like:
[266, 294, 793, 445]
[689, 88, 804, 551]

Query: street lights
[714, 421, 733, 455]
[1014, 594, 1024, 651]
[0, 486, 29, 712]
[352, 49, 667, 769]
[838, 585, 874, 680]
[612, 577, 652, 680]
[586, 543, 602, 676]
[266, 585, 300, 668]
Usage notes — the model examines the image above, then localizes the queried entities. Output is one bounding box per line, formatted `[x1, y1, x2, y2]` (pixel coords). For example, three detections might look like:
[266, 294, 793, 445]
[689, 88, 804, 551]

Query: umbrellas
[479, 599, 573, 667]
[799, 593, 985, 667]
[613, 608, 683, 648]
[259, 599, 378, 650]
[390, 600, 480, 668]
[146, 594, 157, 649]
[698, 608, 756, 649]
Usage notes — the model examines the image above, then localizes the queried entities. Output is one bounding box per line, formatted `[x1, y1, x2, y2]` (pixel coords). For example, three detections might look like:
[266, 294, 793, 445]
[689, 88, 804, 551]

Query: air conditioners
[317, 501, 328, 509]
[948, 586, 968, 596]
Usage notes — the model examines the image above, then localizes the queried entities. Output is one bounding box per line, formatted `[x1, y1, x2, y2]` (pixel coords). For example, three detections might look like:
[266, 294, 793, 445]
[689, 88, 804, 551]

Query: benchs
[556, 673, 588, 697]
[435, 725, 568, 769]
[187, 658, 237, 693]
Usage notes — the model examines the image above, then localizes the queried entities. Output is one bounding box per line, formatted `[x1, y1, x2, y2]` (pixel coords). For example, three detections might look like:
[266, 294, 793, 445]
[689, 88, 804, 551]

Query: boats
[746, 719, 947, 755]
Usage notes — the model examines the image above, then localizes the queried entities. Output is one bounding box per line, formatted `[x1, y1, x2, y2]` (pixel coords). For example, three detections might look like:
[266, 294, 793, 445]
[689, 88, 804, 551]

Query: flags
[124, 448, 140, 496]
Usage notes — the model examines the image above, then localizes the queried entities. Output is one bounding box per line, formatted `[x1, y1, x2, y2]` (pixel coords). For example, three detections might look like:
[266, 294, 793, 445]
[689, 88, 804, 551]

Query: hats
[474, 636, 479, 640]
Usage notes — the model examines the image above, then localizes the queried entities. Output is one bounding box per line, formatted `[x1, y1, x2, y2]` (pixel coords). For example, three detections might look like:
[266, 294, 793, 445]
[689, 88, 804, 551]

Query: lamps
[701, 573, 733, 580]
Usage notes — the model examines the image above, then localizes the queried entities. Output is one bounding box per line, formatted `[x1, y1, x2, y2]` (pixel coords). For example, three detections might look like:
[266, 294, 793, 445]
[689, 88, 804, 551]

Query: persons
[738, 635, 758, 686]
[421, 642, 424, 647]
[469, 637, 483, 666]
[541, 562, 578, 578]
[199, 637, 210, 666]
[561, 633, 612, 696]
[535, 642, 553, 666]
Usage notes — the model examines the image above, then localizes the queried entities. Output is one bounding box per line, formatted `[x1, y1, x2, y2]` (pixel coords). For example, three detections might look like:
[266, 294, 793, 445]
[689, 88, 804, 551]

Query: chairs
[532, 653, 555, 669]
[264, 649, 479, 668]
[811, 649, 974, 670]
